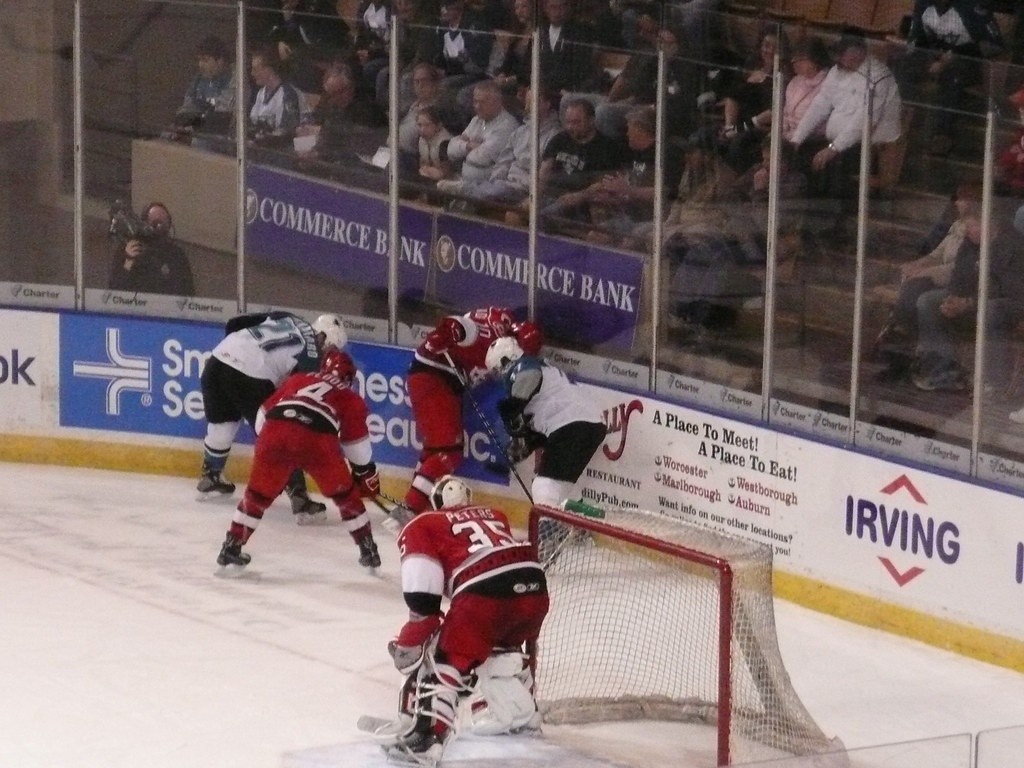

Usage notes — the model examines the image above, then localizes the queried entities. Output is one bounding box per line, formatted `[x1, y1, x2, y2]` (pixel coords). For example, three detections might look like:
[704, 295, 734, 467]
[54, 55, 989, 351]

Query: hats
[829, 30, 866, 52]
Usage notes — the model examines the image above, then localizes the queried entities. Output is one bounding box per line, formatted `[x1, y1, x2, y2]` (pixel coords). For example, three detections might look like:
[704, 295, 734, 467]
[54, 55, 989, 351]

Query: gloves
[350, 463, 380, 500]
[496, 396, 527, 424]
[425, 324, 457, 355]
[506, 431, 534, 465]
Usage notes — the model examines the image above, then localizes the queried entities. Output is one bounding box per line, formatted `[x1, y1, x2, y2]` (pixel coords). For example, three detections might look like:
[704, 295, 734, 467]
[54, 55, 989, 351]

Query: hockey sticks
[461, 384, 533, 505]
[371, 497, 404, 526]
[378, 488, 420, 512]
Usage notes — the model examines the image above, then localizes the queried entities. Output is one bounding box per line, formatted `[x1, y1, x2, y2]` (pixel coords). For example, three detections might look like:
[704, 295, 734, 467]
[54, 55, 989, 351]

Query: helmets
[430, 473, 473, 510]
[309, 313, 347, 350]
[319, 344, 357, 390]
[507, 319, 542, 357]
[485, 337, 524, 375]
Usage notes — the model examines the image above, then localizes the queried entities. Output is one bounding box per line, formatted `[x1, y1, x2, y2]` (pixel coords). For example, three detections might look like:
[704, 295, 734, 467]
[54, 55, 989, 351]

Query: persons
[483, 335, 608, 558]
[388, 305, 542, 525]
[195, 310, 347, 527]
[393, 473, 549, 762]
[217, 345, 383, 569]
[108, 200, 195, 295]
[168, 0, 1024, 423]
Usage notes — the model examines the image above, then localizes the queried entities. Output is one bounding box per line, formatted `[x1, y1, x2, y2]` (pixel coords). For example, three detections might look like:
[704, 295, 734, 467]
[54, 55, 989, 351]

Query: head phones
[141, 201, 171, 236]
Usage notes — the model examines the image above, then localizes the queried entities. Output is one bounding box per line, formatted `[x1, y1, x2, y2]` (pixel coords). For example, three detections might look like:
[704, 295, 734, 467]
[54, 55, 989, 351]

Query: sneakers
[388, 725, 445, 768]
[288, 491, 327, 526]
[537, 519, 556, 564]
[196, 460, 236, 502]
[358, 535, 382, 577]
[213, 531, 252, 578]
[912, 367, 967, 392]
[382, 503, 416, 538]
[557, 523, 596, 547]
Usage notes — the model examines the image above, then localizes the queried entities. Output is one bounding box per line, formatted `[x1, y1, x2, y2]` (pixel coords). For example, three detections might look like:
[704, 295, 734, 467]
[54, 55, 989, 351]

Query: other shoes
[1010, 407, 1024, 423]
[873, 315, 918, 359]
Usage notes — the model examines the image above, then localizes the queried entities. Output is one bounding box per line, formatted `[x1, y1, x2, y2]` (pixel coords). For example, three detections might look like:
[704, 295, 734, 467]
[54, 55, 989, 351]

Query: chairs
[311, 0, 1015, 293]
